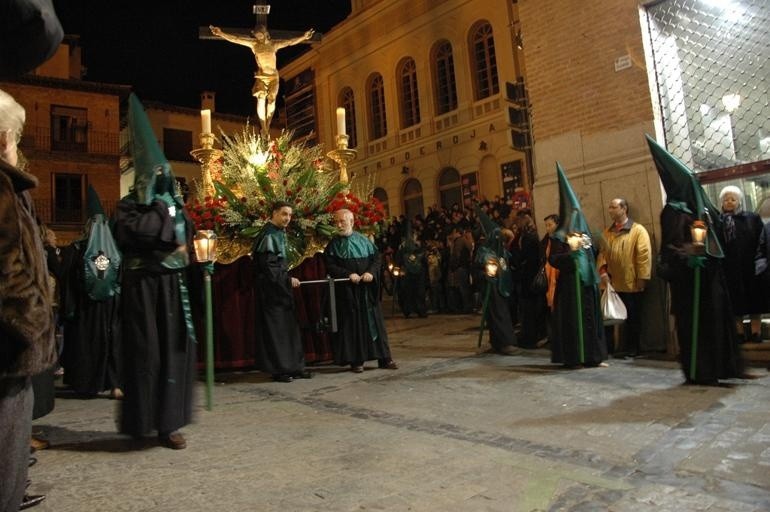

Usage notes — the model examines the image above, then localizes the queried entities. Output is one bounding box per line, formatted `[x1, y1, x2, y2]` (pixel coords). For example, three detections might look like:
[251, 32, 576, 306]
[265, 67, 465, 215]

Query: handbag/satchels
[531, 263, 548, 294]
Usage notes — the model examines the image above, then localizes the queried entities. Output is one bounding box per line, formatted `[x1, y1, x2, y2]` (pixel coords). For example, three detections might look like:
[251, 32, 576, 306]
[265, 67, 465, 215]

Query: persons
[44, 184, 126, 396]
[548, 160, 609, 367]
[755, 199, 768, 276]
[2, 90, 63, 511]
[718, 185, 768, 341]
[210, 23, 315, 141]
[449, 188, 560, 349]
[474, 204, 522, 356]
[250, 201, 314, 382]
[646, 136, 741, 386]
[598, 198, 651, 356]
[375, 208, 456, 317]
[107, 93, 200, 449]
[324, 208, 400, 374]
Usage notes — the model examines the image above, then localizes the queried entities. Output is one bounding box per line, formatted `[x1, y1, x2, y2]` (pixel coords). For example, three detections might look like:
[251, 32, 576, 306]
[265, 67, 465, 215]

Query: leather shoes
[350, 363, 364, 373]
[736, 331, 748, 344]
[750, 333, 762, 342]
[378, 359, 399, 369]
[272, 376, 292, 382]
[158, 430, 186, 449]
[295, 370, 312, 379]
[21, 428, 49, 509]
